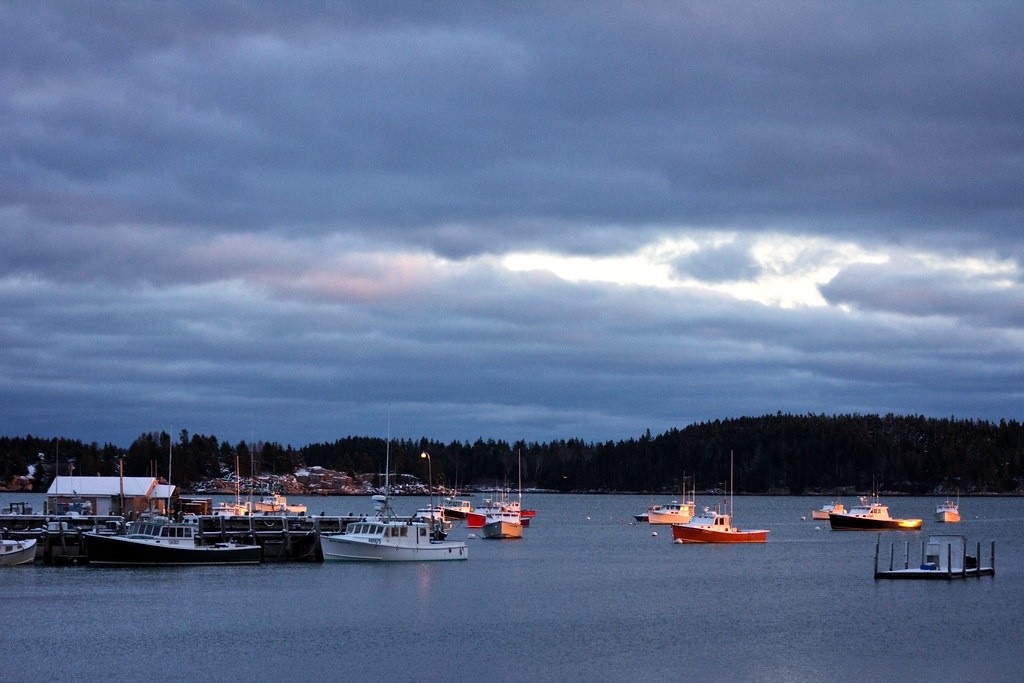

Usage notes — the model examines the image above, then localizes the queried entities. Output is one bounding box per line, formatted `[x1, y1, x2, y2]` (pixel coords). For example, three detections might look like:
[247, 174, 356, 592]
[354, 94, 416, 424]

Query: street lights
[421, 451, 436, 532]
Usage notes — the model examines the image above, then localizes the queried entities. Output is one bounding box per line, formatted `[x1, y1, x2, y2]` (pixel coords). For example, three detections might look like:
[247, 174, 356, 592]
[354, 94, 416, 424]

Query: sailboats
[414, 447, 538, 540]
[318, 402, 470, 562]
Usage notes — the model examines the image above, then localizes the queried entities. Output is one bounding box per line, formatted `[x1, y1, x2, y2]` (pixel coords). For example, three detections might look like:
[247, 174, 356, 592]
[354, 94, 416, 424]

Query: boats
[79, 420, 263, 567]
[633, 487, 663, 522]
[827, 473, 923, 531]
[932, 489, 962, 523]
[671, 449, 772, 544]
[0, 425, 308, 530]
[0, 526, 38, 568]
[811, 487, 848, 521]
[647, 470, 697, 526]
